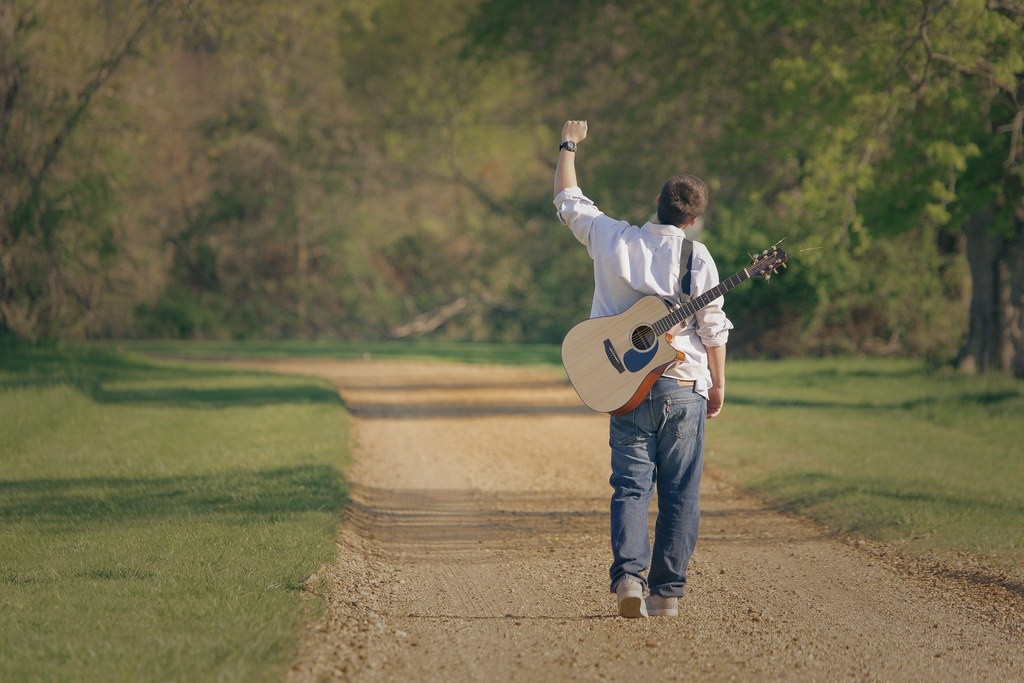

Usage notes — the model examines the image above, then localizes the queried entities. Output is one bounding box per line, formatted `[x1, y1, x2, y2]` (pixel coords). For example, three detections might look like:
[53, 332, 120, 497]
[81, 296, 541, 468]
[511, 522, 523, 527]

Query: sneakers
[616, 579, 649, 619]
[645, 595, 679, 616]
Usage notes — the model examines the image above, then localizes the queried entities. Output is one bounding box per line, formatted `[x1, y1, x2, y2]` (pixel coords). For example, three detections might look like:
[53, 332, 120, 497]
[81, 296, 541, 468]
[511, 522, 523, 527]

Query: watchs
[559, 141, 577, 152]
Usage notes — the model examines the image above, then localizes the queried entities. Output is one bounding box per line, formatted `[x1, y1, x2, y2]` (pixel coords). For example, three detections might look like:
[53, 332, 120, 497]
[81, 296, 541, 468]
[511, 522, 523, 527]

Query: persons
[551, 119, 736, 619]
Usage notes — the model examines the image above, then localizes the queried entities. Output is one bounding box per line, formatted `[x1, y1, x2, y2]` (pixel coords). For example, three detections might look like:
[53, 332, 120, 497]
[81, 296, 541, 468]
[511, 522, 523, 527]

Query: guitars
[561, 235, 824, 416]
